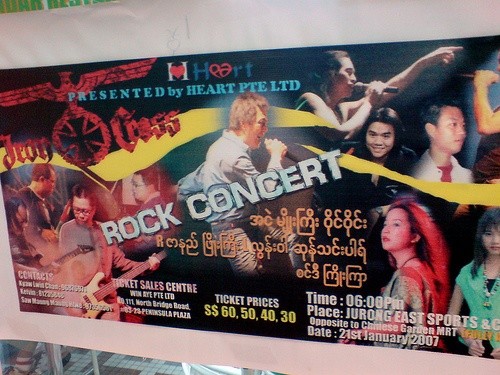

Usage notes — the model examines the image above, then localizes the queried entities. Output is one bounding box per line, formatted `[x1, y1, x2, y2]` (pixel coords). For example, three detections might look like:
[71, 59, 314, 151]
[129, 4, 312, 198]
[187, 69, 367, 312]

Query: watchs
[375, 206, 383, 217]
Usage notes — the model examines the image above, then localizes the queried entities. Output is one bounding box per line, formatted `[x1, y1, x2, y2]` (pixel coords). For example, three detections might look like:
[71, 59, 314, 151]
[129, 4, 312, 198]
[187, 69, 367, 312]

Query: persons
[5, 47, 500, 320]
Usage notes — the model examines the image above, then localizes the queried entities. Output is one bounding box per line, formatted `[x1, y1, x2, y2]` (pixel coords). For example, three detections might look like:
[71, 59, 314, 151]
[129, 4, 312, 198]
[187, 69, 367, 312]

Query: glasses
[71, 208, 93, 216]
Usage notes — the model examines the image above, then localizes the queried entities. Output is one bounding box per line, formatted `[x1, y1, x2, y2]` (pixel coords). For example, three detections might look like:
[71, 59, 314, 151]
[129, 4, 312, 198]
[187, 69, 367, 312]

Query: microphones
[458, 75, 499, 82]
[355, 82, 399, 94]
[285, 152, 299, 162]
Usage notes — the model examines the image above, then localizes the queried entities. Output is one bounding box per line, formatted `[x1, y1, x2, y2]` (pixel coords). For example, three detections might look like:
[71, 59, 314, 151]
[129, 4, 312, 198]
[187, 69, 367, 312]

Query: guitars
[79, 246, 166, 319]
[25, 244, 95, 274]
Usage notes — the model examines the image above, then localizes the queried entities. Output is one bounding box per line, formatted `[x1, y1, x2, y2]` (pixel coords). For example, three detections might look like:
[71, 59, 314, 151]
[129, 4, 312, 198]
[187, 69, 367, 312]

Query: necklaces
[482, 260, 500, 306]
[399, 256, 418, 266]
